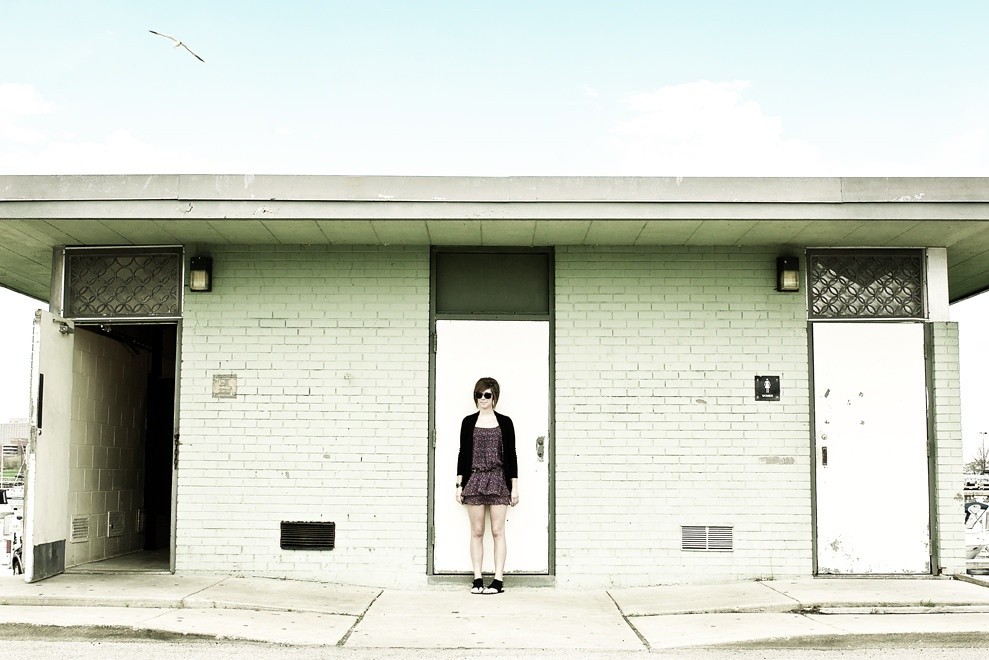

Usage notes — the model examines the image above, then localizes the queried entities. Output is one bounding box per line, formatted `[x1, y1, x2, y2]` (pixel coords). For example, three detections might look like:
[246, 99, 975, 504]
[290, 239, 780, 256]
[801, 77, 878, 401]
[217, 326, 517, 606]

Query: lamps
[190, 255, 212, 291]
[776, 256, 801, 292]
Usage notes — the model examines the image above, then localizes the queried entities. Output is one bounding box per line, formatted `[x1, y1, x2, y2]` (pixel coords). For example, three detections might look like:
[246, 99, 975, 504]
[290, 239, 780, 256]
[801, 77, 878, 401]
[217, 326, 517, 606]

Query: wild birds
[149, 30, 204, 63]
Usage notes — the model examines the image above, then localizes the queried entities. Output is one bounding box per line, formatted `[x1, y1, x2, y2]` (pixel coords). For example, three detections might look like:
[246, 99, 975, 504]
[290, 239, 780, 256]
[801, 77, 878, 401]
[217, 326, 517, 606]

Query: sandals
[481, 579, 505, 594]
[471, 578, 484, 594]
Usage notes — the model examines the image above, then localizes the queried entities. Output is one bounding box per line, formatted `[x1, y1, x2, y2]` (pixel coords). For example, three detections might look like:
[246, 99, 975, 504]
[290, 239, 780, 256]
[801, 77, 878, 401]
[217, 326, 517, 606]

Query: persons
[456, 377, 519, 594]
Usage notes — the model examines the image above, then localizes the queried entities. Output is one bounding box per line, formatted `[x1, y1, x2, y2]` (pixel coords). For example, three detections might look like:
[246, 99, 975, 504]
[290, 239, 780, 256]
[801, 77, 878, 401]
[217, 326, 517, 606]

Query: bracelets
[456, 482, 464, 488]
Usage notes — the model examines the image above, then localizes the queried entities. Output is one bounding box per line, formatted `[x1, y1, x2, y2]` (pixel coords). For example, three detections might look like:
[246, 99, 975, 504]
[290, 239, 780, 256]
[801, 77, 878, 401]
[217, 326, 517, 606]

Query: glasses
[476, 391, 493, 399]
